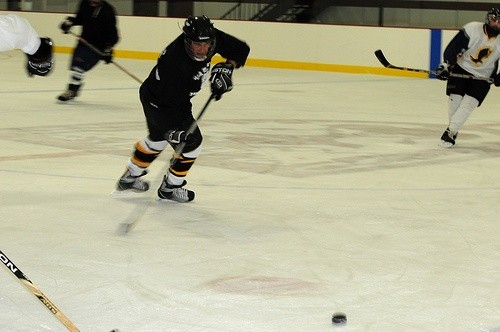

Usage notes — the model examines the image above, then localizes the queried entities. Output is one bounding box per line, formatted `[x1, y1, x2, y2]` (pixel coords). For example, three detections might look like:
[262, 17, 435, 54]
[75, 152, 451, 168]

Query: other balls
[331, 314, 347, 323]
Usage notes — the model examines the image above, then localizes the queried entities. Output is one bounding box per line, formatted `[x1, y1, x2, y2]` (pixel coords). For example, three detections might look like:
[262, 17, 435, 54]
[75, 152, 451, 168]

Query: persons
[111, 15, 251, 204]
[56, 0, 120, 100]
[435, 8, 500, 149]
[0, 13, 53, 78]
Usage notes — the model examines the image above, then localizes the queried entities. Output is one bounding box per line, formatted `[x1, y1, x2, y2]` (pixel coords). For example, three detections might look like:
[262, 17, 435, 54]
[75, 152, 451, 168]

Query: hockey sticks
[109, 93, 215, 237]
[68, 30, 144, 84]
[0, 252, 119, 332]
[374, 49, 496, 85]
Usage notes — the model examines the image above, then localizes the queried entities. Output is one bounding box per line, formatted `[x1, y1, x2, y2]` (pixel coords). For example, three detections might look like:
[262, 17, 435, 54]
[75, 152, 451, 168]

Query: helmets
[485, 7, 500, 38]
[183, 14, 217, 62]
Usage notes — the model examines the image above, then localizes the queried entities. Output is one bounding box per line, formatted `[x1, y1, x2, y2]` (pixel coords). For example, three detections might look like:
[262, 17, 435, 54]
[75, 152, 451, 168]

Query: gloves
[494, 73, 500, 87]
[27, 38, 53, 76]
[209, 62, 234, 101]
[60, 20, 73, 35]
[103, 48, 112, 64]
[435, 63, 451, 80]
[165, 128, 197, 147]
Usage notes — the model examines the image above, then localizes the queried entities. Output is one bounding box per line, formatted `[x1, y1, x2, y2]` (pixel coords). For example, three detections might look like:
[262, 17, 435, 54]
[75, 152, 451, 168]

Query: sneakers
[57, 83, 81, 103]
[155, 174, 195, 204]
[438, 127, 458, 148]
[111, 168, 149, 195]
